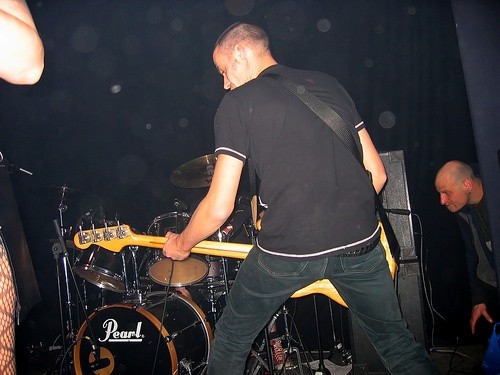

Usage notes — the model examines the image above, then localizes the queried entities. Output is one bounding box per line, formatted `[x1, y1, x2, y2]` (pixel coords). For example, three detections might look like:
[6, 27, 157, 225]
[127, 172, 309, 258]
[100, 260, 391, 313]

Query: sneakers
[273, 344, 294, 370]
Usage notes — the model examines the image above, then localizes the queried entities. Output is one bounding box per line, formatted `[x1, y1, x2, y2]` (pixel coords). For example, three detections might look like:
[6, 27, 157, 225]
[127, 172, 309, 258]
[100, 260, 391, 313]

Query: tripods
[251, 303, 317, 375]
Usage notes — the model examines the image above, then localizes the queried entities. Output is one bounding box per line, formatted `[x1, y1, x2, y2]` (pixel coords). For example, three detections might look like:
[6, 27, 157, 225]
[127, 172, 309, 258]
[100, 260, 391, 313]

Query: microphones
[175, 198, 188, 211]
[88, 358, 110, 371]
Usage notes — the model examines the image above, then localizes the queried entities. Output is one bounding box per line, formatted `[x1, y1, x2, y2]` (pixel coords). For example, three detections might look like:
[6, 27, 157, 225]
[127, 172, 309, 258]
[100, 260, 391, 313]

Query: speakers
[348, 149, 425, 367]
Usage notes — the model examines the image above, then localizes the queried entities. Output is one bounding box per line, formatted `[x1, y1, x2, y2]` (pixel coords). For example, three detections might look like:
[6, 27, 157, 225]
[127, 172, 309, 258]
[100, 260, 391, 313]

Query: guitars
[74, 213, 350, 308]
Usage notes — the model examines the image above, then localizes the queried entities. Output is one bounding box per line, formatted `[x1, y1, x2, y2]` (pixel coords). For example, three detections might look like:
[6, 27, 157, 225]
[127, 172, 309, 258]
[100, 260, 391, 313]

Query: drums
[72, 216, 148, 293]
[73, 291, 213, 375]
[198, 256, 228, 284]
[145, 211, 210, 286]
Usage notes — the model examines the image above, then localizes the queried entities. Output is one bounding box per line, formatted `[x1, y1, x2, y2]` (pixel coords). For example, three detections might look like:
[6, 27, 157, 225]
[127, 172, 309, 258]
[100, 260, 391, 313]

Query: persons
[183, 218, 293, 370]
[0, 0, 44, 375]
[435, 160, 499, 333]
[162, 22, 439, 375]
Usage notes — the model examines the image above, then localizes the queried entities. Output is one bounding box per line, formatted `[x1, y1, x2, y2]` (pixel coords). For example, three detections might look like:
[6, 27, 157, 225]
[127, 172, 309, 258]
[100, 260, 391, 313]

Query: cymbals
[170, 154, 218, 189]
